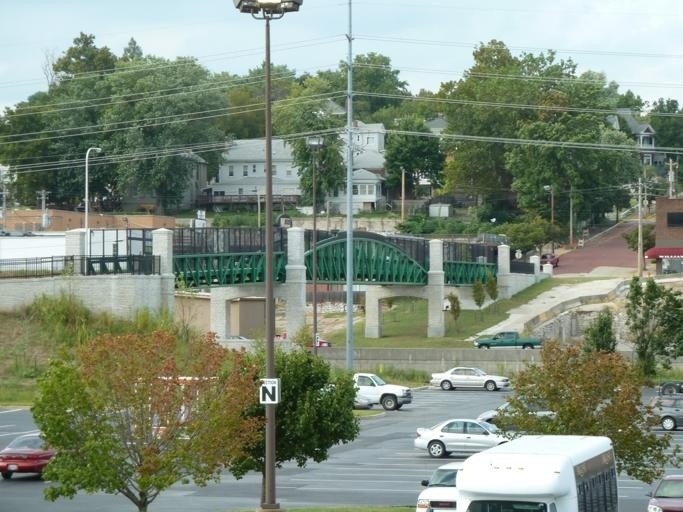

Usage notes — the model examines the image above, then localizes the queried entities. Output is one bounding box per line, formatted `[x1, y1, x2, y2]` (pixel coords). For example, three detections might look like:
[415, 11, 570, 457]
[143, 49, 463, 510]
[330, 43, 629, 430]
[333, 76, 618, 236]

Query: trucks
[133, 375, 220, 445]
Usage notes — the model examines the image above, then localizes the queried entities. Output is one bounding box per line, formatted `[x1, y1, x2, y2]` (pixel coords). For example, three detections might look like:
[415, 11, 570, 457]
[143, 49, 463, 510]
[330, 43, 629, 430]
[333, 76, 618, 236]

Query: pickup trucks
[217, 334, 255, 343]
[324, 371, 413, 413]
[473, 330, 543, 350]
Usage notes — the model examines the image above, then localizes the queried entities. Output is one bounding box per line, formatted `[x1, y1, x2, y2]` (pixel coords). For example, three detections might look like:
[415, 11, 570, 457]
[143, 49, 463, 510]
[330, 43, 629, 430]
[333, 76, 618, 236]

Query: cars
[429, 366, 512, 392]
[1, 433, 55, 479]
[477, 396, 556, 424]
[646, 474, 683, 511]
[540, 253, 560, 268]
[414, 418, 522, 457]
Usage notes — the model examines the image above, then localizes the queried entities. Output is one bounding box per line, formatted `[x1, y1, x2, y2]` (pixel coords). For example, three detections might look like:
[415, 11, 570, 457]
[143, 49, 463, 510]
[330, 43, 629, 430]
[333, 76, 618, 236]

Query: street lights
[543, 184, 554, 254]
[665, 158, 678, 199]
[398, 166, 406, 224]
[305, 135, 325, 355]
[83, 145, 103, 275]
[251, 186, 261, 235]
[230, 0, 301, 510]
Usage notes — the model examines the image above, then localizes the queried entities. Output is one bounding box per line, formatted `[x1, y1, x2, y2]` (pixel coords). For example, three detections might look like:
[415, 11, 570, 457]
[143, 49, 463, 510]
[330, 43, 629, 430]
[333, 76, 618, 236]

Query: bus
[456, 433, 619, 511]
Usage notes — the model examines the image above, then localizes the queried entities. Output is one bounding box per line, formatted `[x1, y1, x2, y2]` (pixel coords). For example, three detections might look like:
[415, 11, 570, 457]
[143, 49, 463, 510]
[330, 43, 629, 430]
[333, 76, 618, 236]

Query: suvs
[655, 380, 683, 396]
[646, 394, 683, 431]
[415, 462, 460, 512]
[274, 332, 330, 347]
